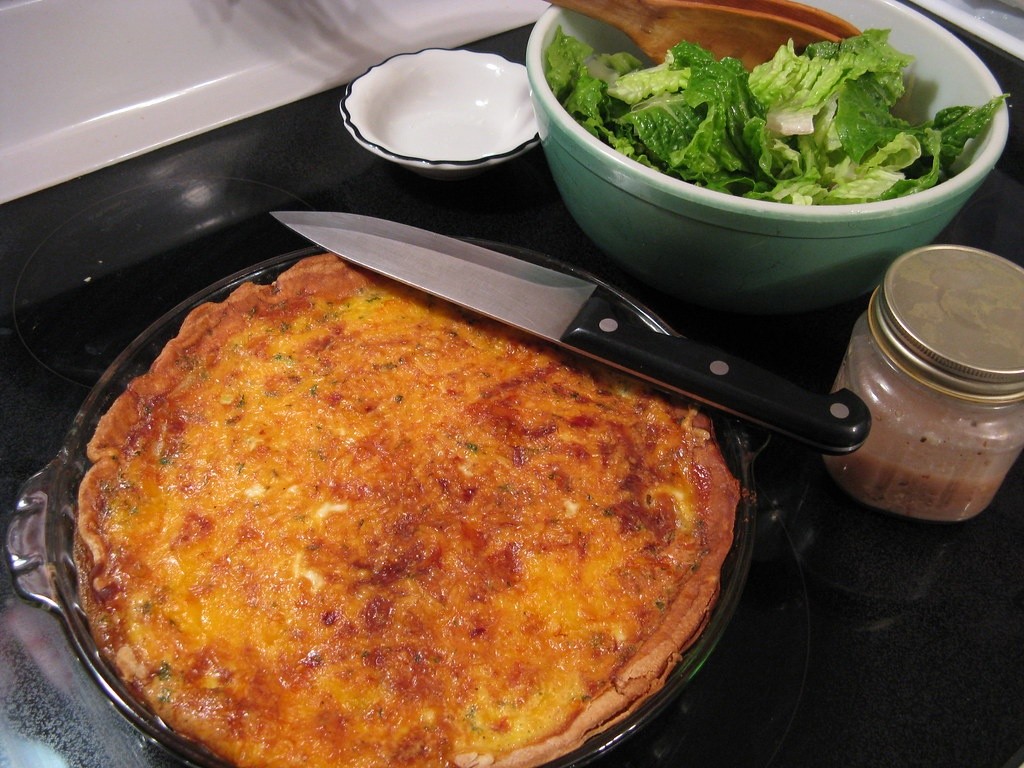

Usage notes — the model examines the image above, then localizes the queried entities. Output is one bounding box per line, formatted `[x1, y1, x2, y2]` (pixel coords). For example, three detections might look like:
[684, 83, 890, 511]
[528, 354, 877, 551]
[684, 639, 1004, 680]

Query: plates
[912, 1, 1024, 64]
[0, 235, 775, 768]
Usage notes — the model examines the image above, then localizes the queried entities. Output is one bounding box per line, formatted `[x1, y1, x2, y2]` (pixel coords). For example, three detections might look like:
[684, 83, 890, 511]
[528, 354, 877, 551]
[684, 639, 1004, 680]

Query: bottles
[821, 244, 1024, 523]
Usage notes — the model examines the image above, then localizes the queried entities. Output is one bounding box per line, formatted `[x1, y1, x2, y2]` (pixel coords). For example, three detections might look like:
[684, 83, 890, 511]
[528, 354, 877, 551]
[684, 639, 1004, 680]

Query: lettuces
[539, 27, 1010, 208]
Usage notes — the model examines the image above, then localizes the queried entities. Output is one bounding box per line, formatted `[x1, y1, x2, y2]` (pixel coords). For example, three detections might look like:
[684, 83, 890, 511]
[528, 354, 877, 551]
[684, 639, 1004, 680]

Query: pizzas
[70, 251, 735, 768]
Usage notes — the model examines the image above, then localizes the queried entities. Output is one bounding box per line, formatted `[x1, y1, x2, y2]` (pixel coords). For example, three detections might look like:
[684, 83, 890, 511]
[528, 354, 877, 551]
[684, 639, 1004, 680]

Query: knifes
[266, 210, 872, 457]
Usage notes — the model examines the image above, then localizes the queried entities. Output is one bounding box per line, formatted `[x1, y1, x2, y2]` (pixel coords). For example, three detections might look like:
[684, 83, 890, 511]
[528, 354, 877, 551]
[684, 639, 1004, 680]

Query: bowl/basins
[338, 47, 540, 181]
[527, 0, 1009, 315]
